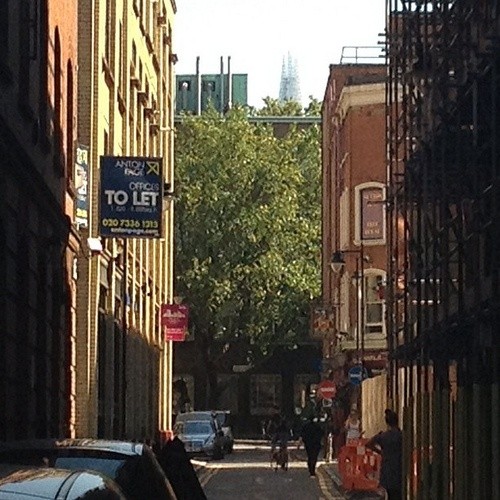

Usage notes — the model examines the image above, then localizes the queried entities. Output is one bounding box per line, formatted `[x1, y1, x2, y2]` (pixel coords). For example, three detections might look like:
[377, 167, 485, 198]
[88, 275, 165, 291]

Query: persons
[297, 412, 329, 480]
[367, 409, 403, 500]
[341, 407, 367, 443]
[265, 412, 292, 472]
[208, 408, 220, 423]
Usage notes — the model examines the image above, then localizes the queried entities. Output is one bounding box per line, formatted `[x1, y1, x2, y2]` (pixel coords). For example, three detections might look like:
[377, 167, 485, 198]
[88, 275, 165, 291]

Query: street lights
[331, 247, 367, 382]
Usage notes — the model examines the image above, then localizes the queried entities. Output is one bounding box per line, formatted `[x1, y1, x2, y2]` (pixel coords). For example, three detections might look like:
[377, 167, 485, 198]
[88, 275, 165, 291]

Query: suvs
[0, 435, 178, 500]
[175, 410, 224, 444]
[214, 411, 233, 455]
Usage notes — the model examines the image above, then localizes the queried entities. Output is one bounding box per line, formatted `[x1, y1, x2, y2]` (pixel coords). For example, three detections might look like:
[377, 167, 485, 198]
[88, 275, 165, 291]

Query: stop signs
[320, 381, 336, 398]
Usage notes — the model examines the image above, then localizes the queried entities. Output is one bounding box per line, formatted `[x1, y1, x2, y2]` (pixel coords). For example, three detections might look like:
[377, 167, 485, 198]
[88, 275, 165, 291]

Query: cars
[1, 464, 135, 499]
[173, 422, 224, 462]
